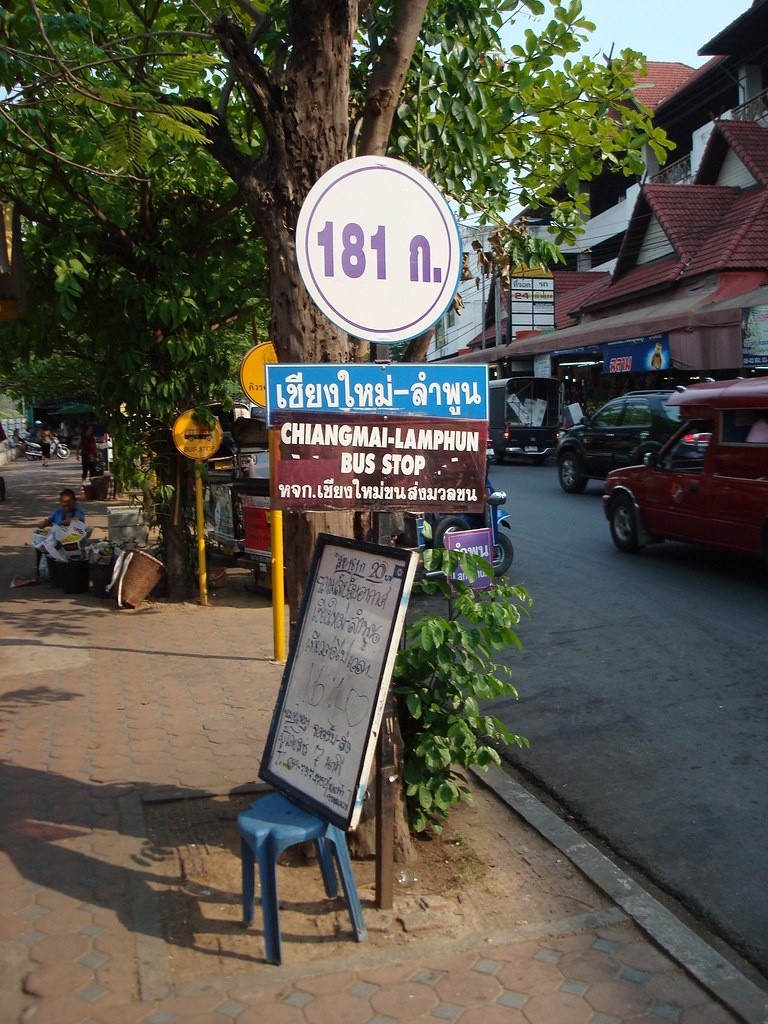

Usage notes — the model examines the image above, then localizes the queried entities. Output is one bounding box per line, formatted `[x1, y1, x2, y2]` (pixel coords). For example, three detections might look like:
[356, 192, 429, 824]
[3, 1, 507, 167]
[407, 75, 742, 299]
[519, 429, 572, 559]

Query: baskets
[114, 547, 167, 609]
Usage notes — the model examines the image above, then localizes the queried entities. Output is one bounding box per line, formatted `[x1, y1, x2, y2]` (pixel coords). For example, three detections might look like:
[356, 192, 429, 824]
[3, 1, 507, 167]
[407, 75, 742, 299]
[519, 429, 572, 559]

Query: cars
[601, 376, 768, 554]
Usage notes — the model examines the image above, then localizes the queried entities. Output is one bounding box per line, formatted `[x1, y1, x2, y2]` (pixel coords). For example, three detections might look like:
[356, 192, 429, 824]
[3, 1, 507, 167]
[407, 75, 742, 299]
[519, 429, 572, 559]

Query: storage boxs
[506, 393, 548, 427]
[106, 505, 147, 548]
[563, 402, 585, 427]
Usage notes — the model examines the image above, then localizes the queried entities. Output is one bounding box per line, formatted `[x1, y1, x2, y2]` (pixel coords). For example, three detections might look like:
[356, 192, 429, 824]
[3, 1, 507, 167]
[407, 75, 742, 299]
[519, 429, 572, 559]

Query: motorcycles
[22, 434, 70, 461]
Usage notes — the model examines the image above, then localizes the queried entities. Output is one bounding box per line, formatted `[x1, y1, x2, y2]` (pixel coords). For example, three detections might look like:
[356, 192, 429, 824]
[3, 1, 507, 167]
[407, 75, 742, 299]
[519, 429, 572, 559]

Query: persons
[76, 425, 99, 481]
[13, 429, 27, 456]
[40, 423, 54, 467]
[37, 490, 85, 530]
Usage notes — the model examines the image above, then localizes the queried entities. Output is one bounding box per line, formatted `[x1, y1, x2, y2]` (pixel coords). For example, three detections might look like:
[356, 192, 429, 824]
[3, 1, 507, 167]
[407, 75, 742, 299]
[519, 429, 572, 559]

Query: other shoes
[42, 464, 50, 467]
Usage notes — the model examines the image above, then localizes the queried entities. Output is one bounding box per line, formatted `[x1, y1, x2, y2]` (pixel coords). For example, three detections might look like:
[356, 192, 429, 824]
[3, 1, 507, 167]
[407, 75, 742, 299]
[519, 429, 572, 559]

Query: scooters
[485, 376, 564, 464]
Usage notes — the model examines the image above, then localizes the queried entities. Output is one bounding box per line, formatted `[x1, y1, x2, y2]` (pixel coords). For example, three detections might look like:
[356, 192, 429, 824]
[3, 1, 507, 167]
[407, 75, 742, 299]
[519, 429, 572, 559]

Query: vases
[45, 555, 64, 589]
[69, 559, 90, 595]
[91, 563, 113, 599]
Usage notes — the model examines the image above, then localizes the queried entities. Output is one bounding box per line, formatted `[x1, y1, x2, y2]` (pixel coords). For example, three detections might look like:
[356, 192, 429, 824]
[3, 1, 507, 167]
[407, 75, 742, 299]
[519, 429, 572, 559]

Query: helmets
[35, 420, 42, 424]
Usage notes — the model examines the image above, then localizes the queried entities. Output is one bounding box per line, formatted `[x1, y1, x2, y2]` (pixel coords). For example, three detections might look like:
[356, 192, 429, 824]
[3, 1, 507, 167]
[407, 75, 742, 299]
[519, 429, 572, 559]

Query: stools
[236, 791, 366, 967]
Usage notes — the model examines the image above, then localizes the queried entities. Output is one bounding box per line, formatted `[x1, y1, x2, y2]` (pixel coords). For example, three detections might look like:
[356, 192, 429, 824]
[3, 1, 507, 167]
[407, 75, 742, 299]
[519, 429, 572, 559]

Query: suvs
[558, 389, 706, 493]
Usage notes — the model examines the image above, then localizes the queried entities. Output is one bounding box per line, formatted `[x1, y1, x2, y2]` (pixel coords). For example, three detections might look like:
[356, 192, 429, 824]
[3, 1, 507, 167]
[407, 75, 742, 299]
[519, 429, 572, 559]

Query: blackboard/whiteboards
[257, 531, 422, 837]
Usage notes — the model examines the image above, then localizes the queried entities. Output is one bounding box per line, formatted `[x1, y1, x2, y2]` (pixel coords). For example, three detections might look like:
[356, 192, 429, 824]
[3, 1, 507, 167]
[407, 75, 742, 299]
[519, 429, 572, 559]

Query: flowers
[33, 523, 140, 561]
